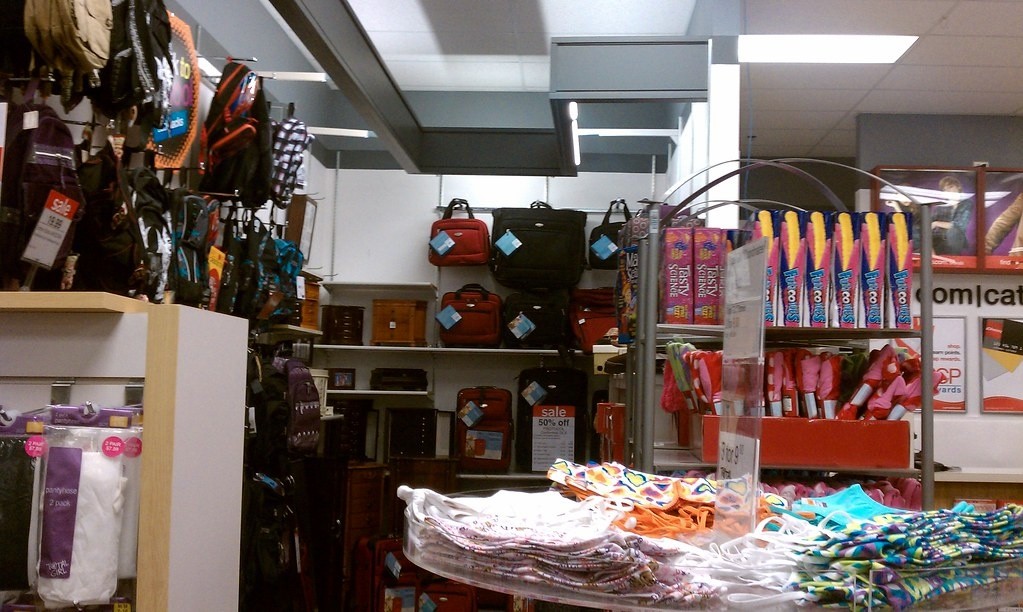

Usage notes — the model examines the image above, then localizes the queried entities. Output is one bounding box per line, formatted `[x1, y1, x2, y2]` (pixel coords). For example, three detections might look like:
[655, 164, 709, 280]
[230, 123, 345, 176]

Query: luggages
[456, 385, 514, 472]
[351, 528, 477, 612]
[515, 355, 588, 468]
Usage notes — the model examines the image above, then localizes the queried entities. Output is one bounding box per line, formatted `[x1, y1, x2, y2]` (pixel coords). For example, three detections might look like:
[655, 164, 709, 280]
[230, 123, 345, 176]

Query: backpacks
[0, 0, 303, 322]
[241, 346, 322, 584]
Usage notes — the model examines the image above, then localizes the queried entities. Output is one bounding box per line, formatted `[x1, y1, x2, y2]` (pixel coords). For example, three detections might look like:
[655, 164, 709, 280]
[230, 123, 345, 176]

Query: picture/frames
[326, 367, 356, 390]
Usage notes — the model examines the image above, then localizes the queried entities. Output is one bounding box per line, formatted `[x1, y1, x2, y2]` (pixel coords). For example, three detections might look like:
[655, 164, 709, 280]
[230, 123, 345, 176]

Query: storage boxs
[658, 208, 913, 329]
[676, 408, 908, 471]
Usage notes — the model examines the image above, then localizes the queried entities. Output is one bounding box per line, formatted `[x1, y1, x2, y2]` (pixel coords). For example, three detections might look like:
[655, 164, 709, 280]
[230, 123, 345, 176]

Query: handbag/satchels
[440, 283, 502, 347]
[428, 198, 490, 266]
[502, 288, 569, 349]
[589, 200, 632, 270]
[569, 287, 617, 354]
[490, 201, 587, 291]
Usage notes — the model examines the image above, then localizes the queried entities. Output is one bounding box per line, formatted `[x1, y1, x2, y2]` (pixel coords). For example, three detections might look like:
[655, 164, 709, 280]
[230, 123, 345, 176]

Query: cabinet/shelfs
[387, 458, 450, 536]
[268, 323, 343, 420]
[624, 158, 933, 514]
[313, 281, 589, 480]
[311, 458, 388, 612]
[300, 282, 322, 330]
[369, 298, 428, 349]
